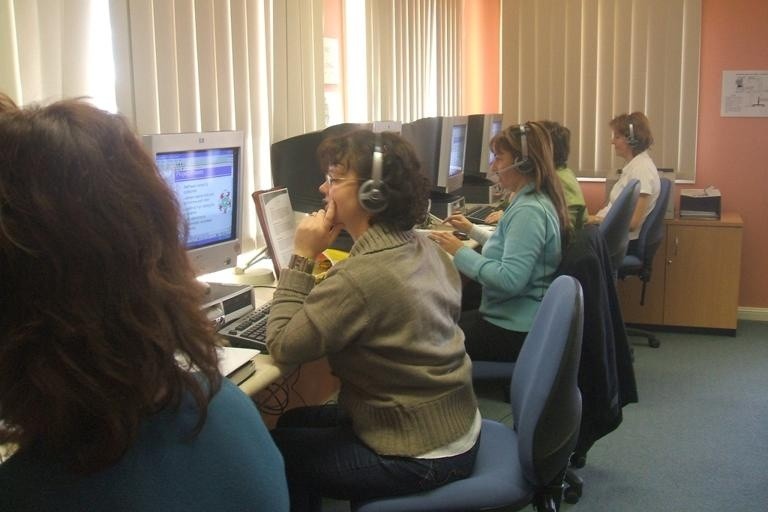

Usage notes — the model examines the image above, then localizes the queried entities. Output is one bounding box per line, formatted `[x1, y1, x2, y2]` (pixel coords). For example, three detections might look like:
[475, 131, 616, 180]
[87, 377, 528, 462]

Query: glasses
[326, 173, 368, 186]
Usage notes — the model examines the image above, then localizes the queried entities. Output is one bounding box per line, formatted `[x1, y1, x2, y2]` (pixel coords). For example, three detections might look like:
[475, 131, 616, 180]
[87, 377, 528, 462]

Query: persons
[484, 120, 583, 241]
[2, 88, 291, 511]
[429, 123, 566, 361]
[265, 130, 483, 511]
[585, 110, 662, 253]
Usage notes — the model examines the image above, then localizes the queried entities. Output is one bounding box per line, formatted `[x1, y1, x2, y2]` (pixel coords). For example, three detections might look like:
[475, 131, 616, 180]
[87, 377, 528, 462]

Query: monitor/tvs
[403, 116, 468, 195]
[466, 113, 505, 180]
[273, 121, 401, 239]
[142, 129, 244, 278]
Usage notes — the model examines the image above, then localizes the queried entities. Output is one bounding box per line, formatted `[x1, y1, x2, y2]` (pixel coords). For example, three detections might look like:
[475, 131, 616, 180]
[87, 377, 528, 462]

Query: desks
[193, 225, 497, 396]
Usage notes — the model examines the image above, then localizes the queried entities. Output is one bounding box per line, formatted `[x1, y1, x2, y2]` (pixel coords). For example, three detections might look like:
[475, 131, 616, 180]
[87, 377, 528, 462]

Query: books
[227, 359, 256, 388]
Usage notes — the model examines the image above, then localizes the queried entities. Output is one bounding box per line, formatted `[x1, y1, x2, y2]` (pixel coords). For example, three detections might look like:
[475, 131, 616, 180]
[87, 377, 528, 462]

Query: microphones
[496, 163, 522, 175]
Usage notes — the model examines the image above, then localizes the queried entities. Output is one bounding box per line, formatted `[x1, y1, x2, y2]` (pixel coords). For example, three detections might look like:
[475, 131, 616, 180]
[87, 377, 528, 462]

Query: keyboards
[463, 206, 497, 225]
[216, 297, 272, 352]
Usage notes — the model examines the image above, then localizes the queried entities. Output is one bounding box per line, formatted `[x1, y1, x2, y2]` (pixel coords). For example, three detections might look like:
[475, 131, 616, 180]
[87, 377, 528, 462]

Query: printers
[606, 166, 676, 220]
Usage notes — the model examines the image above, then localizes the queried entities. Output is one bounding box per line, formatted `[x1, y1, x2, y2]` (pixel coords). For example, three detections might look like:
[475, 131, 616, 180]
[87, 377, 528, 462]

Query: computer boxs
[465, 182, 502, 205]
[197, 281, 254, 334]
[430, 196, 465, 218]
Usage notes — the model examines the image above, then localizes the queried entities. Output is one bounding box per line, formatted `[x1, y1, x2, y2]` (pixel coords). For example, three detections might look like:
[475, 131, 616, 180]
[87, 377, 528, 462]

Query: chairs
[472, 223, 601, 383]
[351, 275, 584, 512]
[596, 178, 641, 277]
[618, 178, 672, 347]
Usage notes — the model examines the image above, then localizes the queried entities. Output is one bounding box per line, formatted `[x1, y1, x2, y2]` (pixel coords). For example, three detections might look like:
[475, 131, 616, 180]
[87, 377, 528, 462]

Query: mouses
[453, 230, 469, 240]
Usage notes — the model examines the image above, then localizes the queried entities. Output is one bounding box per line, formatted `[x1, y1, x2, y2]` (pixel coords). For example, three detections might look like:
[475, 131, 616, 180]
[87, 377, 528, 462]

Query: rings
[310, 213, 316, 216]
[434, 239, 440, 244]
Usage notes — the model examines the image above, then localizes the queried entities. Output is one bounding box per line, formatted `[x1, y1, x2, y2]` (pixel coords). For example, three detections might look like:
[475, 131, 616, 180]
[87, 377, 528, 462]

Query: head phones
[627, 121, 639, 149]
[356, 137, 393, 215]
[514, 124, 537, 173]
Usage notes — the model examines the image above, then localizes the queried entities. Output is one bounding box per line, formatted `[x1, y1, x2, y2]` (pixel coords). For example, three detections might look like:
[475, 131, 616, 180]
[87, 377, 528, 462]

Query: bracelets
[286, 253, 315, 275]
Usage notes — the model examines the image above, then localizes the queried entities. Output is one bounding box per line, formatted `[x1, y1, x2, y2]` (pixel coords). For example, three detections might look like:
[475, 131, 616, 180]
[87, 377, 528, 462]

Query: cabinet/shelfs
[616, 212, 743, 336]
